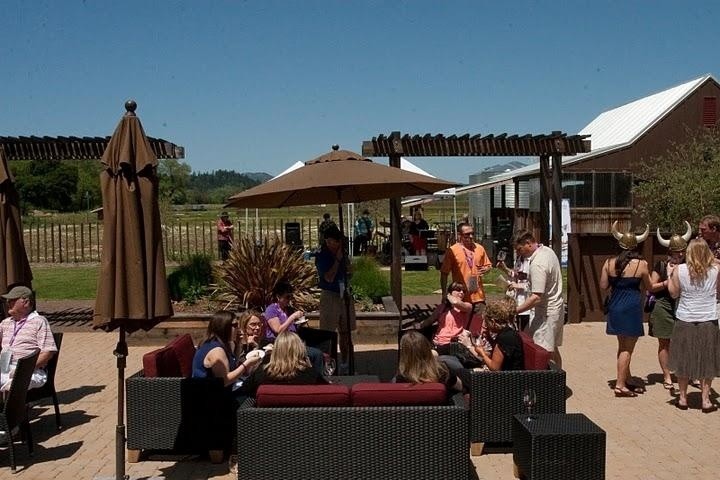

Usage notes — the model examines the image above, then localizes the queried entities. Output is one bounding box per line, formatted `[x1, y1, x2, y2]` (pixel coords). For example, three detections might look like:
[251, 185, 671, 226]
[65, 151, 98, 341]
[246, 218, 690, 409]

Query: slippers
[674, 397, 688, 409]
[702, 402, 718, 413]
[663, 378, 673, 389]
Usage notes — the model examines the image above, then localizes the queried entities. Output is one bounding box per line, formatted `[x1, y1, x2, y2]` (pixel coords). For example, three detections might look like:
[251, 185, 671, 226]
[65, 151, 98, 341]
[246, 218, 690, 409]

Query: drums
[403, 234, 410, 242]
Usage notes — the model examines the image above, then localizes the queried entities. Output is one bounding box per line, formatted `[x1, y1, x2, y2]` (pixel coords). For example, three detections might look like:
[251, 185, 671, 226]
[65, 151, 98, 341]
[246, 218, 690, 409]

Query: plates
[295, 319, 308, 325]
[246, 350, 265, 362]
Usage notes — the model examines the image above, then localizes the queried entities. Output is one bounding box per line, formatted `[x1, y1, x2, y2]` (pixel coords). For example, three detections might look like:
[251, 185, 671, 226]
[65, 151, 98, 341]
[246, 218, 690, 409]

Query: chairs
[0, 348, 41, 473]
[26, 333, 63, 430]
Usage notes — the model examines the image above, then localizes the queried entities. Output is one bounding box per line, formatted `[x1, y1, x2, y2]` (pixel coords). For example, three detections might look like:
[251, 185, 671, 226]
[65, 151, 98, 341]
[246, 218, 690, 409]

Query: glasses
[463, 232, 474, 236]
[452, 286, 465, 293]
[232, 321, 238, 327]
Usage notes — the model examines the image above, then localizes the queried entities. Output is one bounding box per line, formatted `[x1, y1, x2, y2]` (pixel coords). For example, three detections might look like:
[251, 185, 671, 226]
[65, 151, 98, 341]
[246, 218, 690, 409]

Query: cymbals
[379, 222, 390, 227]
[377, 231, 387, 238]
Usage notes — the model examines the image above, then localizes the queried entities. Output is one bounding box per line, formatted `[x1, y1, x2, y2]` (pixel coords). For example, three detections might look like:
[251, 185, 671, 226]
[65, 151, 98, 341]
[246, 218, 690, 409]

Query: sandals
[615, 386, 636, 397]
[625, 380, 644, 392]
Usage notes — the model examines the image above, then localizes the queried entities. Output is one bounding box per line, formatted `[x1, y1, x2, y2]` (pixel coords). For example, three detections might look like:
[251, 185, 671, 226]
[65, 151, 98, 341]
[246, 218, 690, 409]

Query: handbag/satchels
[644, 261, 665, 313]
[603, 295, 611, 314]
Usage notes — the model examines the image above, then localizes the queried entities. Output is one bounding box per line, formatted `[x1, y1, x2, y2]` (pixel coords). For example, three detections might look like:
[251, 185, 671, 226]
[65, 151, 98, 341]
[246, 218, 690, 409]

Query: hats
[610, 220, 650, 251]
[0, 286, 34, 300]
[220, 212, 229, 217]
[656, 221, 692, 252]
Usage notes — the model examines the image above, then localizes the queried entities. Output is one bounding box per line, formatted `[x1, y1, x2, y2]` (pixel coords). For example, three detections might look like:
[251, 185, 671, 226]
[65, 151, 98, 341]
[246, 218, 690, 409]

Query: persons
[216, 213, 234, 260]
[317, 213, 350, 376]
[355, 210, 374, 248]
[441, 222, 564, 372]
[648, 220, 701, 390]
[408, 210, 429, 256]
[193, 280, 326, 398]
[665, 237, 720, 414]
[697, 214, 720, 260]
[597, 220, 654, 397]
[392, 281, 473, 391]
[0, 285, 59, 448]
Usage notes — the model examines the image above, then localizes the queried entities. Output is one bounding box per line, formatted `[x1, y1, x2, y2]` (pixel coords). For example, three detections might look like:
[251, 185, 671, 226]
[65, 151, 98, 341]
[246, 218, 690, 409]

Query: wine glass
[497, 250, 507, 264]
[325, 358, 336, 384]
[522, 389, 536, 422]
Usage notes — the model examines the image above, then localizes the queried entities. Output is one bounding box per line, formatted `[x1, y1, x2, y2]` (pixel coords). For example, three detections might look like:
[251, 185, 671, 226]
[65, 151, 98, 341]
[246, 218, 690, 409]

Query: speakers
[286, 222, 300, 245]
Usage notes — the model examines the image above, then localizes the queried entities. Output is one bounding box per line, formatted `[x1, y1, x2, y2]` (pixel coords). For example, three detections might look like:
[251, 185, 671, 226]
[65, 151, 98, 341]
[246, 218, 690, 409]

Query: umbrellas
[222, 142, 467, 376]
[88, 99, 177, 480]
[0, 140, 35, 302]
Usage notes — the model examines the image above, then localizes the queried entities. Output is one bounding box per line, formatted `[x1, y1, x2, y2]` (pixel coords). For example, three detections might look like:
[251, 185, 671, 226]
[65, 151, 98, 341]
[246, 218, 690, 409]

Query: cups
[463, 329, 471, 337]
[298, 316, 306, 322]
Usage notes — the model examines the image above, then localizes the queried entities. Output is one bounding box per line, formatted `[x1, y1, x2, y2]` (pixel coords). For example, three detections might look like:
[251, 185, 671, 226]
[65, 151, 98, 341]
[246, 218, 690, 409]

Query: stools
[513, 413, 606, 480]
